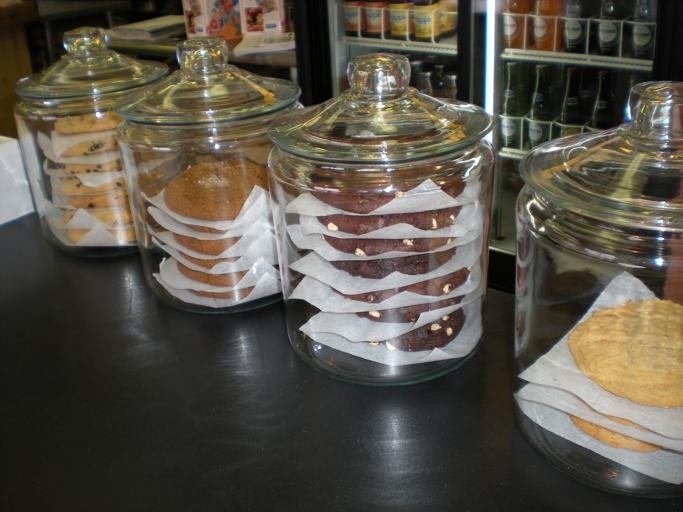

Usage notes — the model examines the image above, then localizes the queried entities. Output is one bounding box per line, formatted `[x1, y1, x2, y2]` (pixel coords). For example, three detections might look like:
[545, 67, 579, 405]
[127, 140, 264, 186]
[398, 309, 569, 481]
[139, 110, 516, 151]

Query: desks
[102, 32, 299, 68]
[0, 208, 681, 512]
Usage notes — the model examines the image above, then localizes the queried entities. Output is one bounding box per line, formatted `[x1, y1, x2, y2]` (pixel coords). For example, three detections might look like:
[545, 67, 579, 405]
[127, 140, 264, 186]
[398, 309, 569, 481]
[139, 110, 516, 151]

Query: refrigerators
[288, 0, 683, 289]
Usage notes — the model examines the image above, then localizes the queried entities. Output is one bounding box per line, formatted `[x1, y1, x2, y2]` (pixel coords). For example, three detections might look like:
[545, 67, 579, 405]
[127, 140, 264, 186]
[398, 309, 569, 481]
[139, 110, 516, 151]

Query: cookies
[309, 124, 470, 352]
[53, 99, 268, 299]
[567, 298, 683, 454]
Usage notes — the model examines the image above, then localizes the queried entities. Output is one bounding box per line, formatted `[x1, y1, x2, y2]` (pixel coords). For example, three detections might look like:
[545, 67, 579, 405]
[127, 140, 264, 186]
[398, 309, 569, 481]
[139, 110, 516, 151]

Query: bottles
[492, 0, 664, 158]
[264, 54, 501, 389]
[11, 25, 169, 261]
[337, 1, 459, 102]
[111, 34, 312, 316]
[509, 77, 683, 501]
